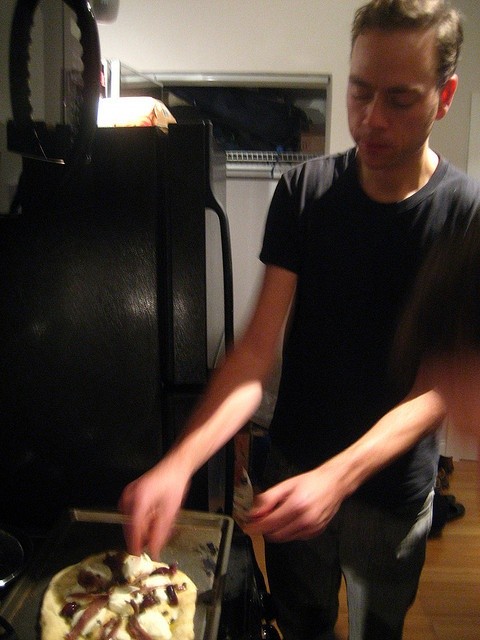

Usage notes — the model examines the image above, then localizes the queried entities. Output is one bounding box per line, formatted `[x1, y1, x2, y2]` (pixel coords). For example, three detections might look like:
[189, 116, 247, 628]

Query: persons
[121, 0, 480, 637]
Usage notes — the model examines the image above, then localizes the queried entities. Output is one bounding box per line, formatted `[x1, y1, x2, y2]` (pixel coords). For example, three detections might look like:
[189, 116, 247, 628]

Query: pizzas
[39, 550, 197, 640]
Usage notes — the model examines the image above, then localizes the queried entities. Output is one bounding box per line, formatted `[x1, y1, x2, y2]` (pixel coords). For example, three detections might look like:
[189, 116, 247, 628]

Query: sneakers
[434, 478, 442, 494]
[438, 467, 449, 488]
[447, 504, 465, 520]
[444, 494, 455, 506]
[439, 455, 454, 474]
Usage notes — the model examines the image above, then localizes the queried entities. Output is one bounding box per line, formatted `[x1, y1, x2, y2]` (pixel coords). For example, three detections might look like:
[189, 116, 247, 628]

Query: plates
[0, 504, 235, 640]
[0, 511, 31, 587]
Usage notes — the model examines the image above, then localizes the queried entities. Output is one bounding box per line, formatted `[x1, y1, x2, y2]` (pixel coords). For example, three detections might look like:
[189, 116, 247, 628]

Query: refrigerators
[1, 116, 237, 523]
